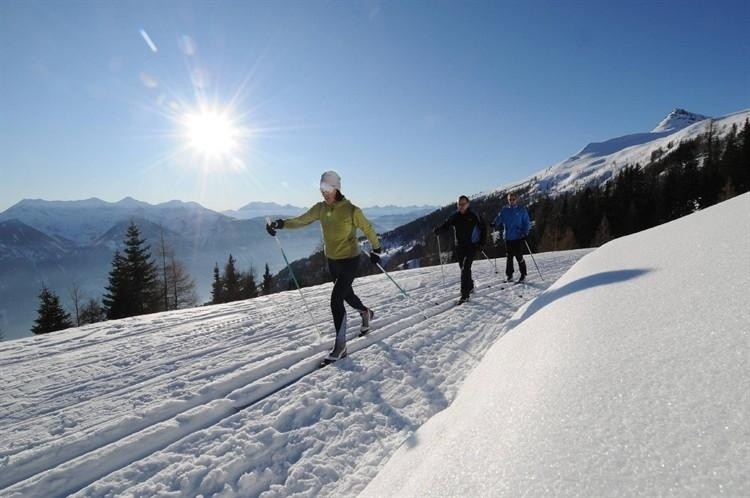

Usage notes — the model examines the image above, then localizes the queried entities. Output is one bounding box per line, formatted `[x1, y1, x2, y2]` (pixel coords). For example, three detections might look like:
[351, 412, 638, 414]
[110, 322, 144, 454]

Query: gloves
[433, 228, 441, 234]
[521, 234, 528, 240]
[369, 250, 382, 264]
[490, 223, 496, 228]
[478, 246, 483, 252]
[266, 218, 284, 236]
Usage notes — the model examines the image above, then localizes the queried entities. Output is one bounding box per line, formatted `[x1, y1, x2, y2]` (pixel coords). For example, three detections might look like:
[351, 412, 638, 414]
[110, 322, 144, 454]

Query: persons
[432, 194, 486, 304]
[265, 169, 381, 363]
[491, 192, 532, 284]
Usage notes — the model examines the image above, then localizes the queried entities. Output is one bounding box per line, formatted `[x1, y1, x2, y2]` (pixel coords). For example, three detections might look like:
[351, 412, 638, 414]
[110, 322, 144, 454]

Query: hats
[319, 170, 341, 190]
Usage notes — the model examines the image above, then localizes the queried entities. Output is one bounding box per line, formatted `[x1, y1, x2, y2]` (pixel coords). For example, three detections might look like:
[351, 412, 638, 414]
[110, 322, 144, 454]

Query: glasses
[457, 202, 467, 207]
[508, 198, 516, 202]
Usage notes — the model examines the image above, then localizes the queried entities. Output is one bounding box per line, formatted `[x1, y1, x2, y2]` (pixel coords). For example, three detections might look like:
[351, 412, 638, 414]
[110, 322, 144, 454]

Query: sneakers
[505, 274, 513, 280]
[519, 274, 526, 282]
[456, 282, 474, 305]
[359, 309, 375, 334]
[324, 341, 350, 362]
